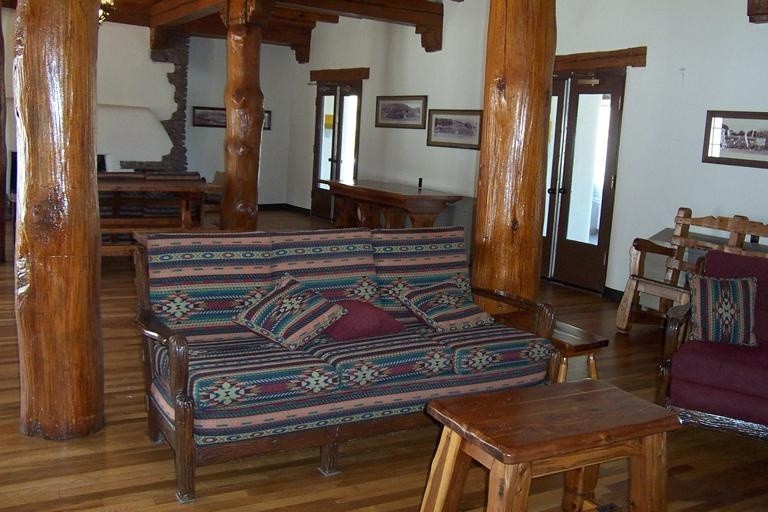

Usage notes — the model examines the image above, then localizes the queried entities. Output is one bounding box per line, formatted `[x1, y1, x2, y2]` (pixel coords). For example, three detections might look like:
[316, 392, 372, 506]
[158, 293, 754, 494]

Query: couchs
[204, 171, 226, 203]
[96, 169, 202, 256]
[132, 225, 570, 505]
[655, 250, 768, 441]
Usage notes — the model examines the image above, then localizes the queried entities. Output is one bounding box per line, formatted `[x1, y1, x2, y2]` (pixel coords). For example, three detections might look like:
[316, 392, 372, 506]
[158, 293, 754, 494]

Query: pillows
[231, 276, 348, 350]
[687, 272, 758, 348]
[397, 281, 495, 335]
[322, 300, 405, 340]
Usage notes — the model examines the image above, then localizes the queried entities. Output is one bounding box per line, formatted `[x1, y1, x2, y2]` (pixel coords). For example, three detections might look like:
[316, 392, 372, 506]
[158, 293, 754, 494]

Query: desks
[331, 180, 464, 228]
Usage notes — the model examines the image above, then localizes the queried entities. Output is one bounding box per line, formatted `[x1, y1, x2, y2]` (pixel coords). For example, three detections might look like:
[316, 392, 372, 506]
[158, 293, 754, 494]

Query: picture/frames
[427, 109, 483, 150]
[375, 95, 427, 129]
[193, 106, 272, 131]
[702, 110, 768, 169]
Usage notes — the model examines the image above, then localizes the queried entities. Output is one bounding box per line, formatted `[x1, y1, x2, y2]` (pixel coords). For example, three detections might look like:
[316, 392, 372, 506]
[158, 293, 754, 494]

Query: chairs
[615, 207, 768, 335]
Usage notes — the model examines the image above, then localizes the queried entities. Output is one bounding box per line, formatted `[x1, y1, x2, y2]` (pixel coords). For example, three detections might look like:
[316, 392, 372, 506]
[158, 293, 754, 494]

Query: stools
[492, 306, 610, 383]
[421, 378, 683, 511]
